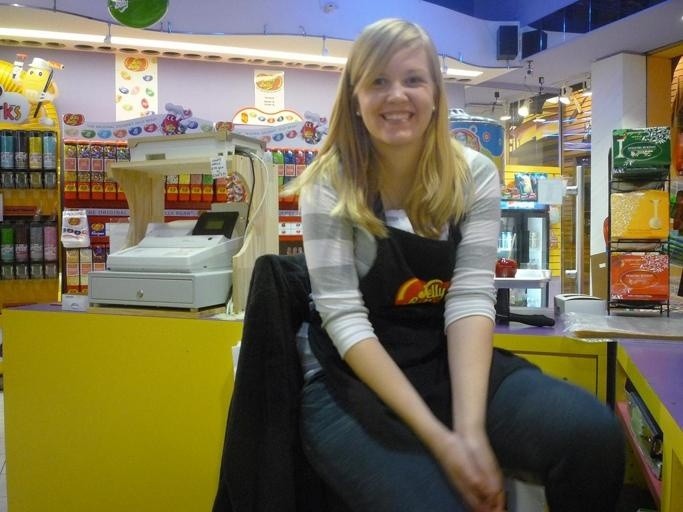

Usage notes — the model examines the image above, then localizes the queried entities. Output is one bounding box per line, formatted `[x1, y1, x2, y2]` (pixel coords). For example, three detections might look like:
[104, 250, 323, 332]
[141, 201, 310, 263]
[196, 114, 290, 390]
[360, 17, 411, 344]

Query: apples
[496, 259, 518, 277]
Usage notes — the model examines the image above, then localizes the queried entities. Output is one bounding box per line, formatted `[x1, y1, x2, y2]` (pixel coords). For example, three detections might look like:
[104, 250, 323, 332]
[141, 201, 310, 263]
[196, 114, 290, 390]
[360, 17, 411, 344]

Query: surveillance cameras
[323, 3, 336, 13]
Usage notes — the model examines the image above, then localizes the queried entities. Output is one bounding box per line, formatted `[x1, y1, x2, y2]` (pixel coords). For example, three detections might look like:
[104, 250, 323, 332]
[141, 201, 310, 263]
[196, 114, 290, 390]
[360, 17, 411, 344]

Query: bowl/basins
[494, 258, 517, 276]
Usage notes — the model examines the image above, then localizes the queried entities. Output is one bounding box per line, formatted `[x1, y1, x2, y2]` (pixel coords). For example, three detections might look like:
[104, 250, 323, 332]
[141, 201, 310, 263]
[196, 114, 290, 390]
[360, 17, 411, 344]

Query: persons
[296, 19, 626, 512]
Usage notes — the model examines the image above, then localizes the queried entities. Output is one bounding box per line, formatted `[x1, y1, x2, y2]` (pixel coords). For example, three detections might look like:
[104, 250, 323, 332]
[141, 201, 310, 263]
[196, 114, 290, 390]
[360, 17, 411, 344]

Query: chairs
[215, 256, 516, 512]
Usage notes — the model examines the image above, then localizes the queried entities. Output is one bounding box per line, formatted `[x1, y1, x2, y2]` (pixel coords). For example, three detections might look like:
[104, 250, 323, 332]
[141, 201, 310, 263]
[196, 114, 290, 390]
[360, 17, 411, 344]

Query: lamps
[517, 74, 592, 117]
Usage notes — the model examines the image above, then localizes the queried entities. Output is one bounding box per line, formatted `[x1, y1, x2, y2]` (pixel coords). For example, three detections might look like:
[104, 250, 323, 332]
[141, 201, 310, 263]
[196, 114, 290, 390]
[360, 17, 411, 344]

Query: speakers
[522, 30, 546, 58]
[497, 25, 518, 60]
